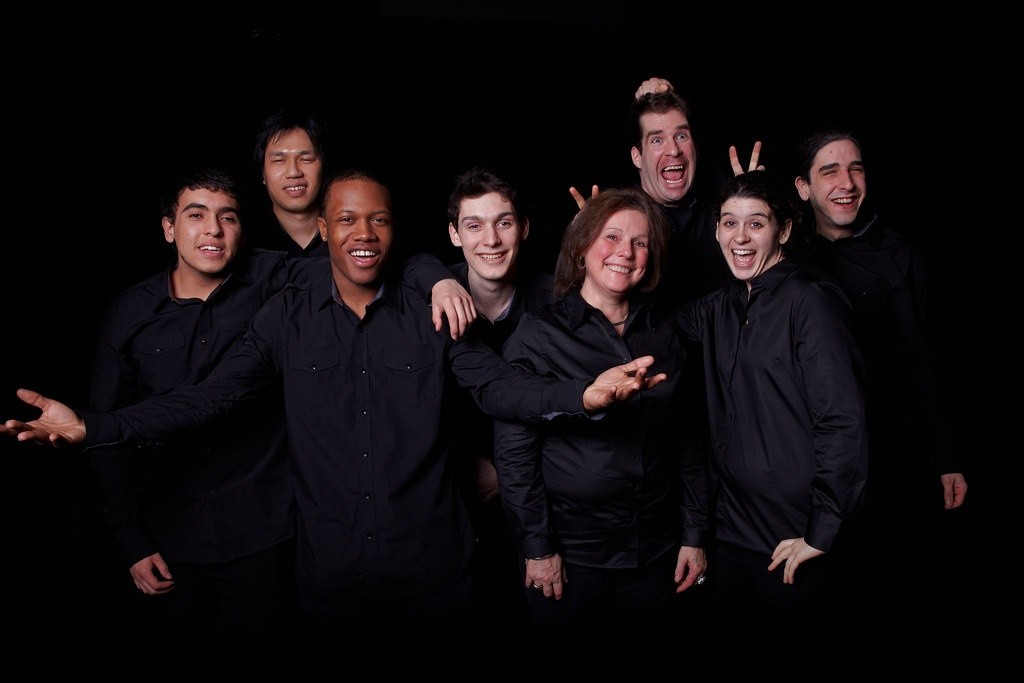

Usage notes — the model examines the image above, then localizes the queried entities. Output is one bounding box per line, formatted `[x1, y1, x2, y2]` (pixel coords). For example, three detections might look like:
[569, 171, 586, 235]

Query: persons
[437, 173, 543, 683]
[0, 170, 669, 682]
[568, 90, 768, 324]
[495, 189, 717, 682]
[568, 170, 878, 681]
[212, 109, 333, 260]
[86, 170, 475, 683]
[633, 77, 969, 682]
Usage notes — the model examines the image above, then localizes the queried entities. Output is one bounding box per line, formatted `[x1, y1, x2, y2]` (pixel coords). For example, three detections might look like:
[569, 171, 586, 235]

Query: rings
[532, 582, 542, 592]
[696, 576, 707, 585]
